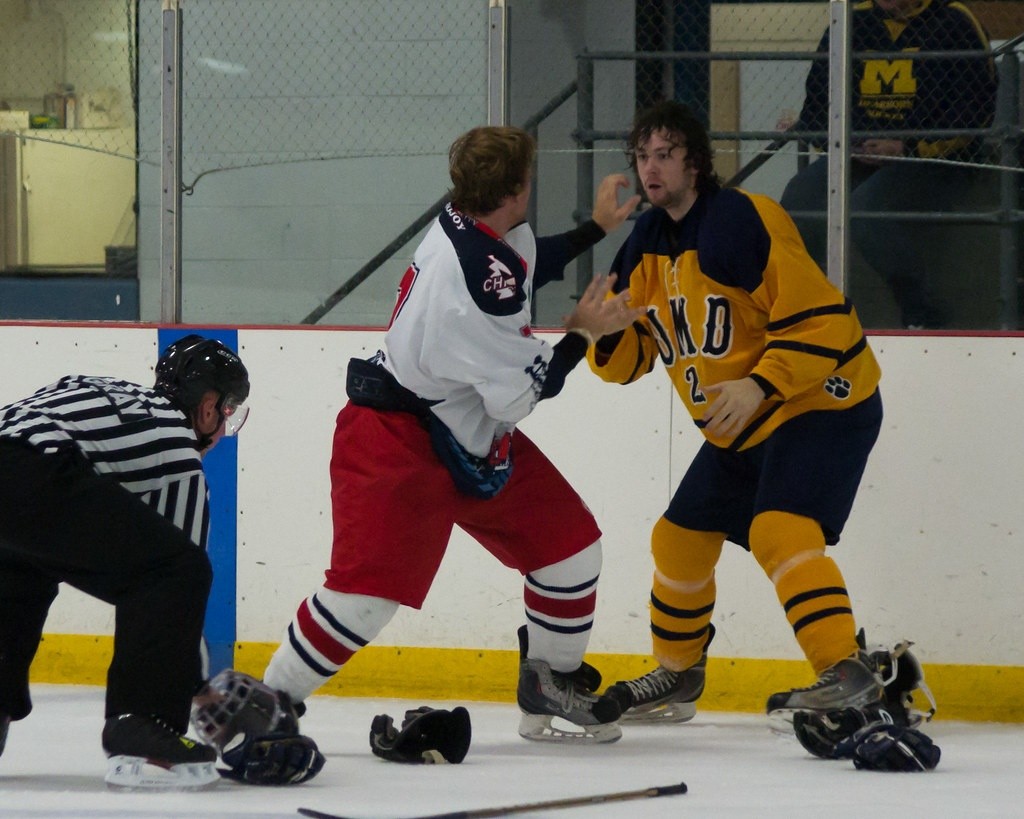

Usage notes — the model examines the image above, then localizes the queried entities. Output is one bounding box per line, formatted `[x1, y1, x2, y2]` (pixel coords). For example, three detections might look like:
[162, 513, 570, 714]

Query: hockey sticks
[298, 783, 686, 819]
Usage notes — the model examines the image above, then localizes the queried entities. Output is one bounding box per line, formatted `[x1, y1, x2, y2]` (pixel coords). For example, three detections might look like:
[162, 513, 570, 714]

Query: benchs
[801, 61, 1024, 331]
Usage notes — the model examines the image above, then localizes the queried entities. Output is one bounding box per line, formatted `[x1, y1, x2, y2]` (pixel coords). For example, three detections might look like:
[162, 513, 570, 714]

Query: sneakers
[610, 657, 708, 726]
[101, 714, 219, 789]
[767, 656, 885, 732]
[520, 659, 626, 744]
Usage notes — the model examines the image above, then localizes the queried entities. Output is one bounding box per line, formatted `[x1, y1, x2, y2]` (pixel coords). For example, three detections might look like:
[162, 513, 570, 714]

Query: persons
[0, 336, 253, 792]
[581, 104, 933, 740]
[221, 125, 647, 755]
[776, 1, 999, 329]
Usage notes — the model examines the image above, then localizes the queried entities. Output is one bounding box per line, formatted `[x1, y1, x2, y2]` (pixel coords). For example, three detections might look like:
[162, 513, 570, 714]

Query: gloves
[216, 734, 325, 785]
[796, 707, 903, 758]
[853, 724, 939, 771]
[372, 705, 472, 762]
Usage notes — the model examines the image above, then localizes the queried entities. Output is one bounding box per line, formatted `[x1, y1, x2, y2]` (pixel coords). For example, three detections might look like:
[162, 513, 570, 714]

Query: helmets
[159, 334, 250, 444]
[859, 634, 937, 722]
[196, 671, 297, 758]
